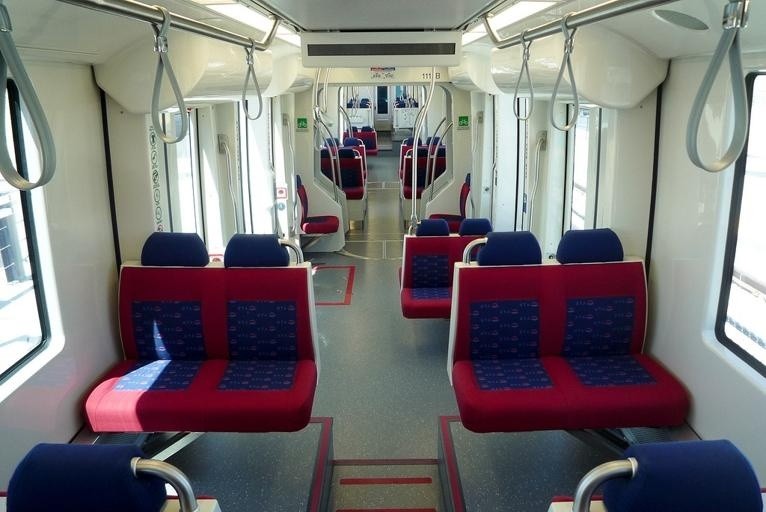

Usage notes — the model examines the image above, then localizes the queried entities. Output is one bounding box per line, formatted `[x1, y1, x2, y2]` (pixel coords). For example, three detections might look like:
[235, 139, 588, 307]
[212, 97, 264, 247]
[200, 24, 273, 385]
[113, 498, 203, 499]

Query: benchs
[399, 136, 446, 199]
[321, 126, 379, 200]
[348, 98, 372, 108]
[399, 219, 492, 318]
[393, 97, 418, 108]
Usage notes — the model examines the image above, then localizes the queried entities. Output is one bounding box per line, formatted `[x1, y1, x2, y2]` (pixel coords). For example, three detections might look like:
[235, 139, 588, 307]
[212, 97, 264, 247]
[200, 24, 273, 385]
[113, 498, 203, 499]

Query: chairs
[429, 172, 470, 233]
[297, 174, 338, 251]
